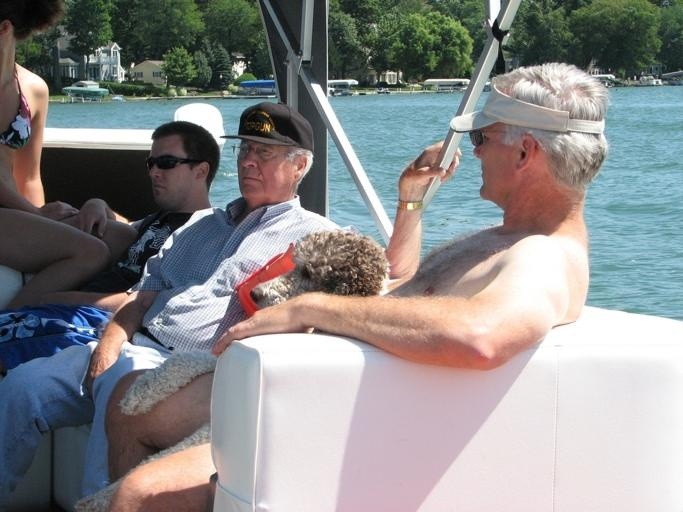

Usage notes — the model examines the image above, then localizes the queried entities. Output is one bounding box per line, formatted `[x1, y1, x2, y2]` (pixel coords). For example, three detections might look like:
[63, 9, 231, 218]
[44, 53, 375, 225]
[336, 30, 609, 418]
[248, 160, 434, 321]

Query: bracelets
[396, 199, 424, 210]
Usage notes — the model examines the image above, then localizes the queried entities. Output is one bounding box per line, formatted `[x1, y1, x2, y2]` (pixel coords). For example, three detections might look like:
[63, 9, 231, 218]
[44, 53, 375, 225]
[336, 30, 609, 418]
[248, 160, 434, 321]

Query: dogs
[74, 230, 392, 512]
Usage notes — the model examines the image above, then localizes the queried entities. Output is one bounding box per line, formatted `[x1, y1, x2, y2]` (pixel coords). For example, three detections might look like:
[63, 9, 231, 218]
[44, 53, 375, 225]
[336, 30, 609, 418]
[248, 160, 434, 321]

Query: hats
[449, 84, 605, 135]
[220, 102, 315, 151]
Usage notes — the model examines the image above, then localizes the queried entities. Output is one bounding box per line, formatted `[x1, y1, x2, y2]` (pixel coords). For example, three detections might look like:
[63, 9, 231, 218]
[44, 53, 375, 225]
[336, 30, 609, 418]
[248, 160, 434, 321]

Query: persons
[0, 0, 138, 311]
[0, 101, 463, 512]
[105, 62, 610, 512]
[0, 120, 221, 377]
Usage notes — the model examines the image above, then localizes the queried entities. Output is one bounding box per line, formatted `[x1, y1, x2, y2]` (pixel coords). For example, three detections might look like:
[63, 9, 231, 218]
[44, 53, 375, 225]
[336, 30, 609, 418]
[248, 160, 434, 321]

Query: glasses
[468, 131, 486, 146]
[231, 143, 296, 157]
[146, 156, 196, 170]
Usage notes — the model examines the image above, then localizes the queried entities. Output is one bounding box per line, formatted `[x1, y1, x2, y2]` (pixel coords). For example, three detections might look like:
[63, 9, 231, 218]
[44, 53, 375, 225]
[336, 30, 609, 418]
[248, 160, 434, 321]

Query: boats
[332, 89, 353, 97]
[377, 88, 391, 95]
[599, 74, 683, 88]
[111, 94, 124, 100]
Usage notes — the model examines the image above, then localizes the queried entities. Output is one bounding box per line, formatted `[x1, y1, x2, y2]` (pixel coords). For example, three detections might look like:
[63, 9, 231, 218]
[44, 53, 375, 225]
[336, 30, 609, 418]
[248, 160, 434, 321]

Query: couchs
[0, 263, 683, 512]
